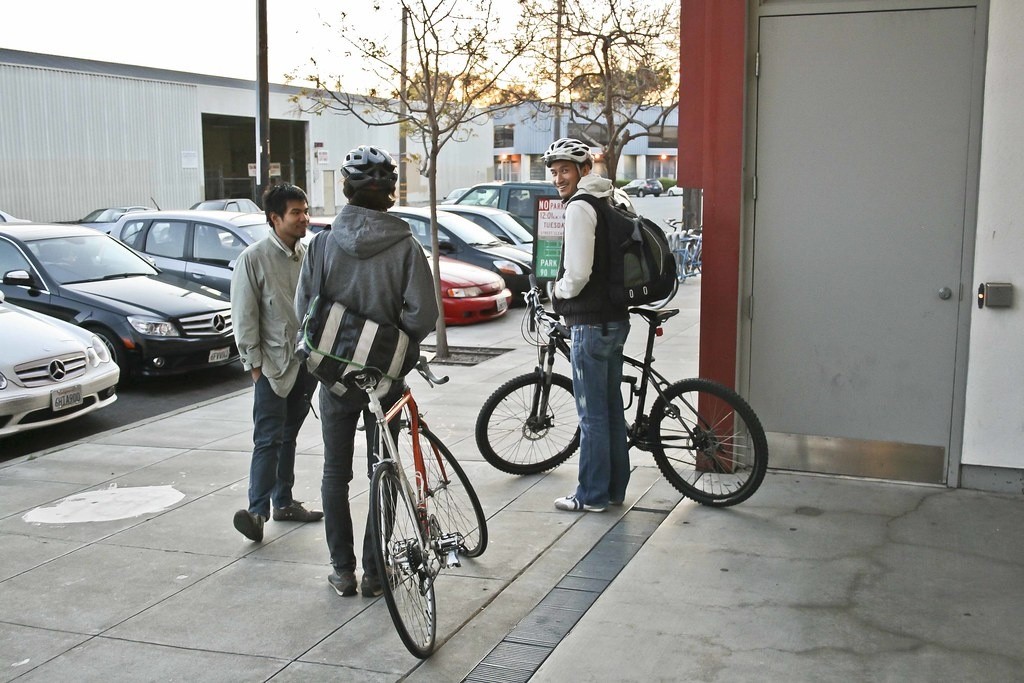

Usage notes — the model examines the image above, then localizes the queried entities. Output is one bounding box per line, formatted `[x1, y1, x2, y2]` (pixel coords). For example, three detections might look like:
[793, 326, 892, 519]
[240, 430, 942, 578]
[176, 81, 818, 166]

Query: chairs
[203, 229, 234, 260]
[39, 244, 84, 283]
[508, 197, 530, 216]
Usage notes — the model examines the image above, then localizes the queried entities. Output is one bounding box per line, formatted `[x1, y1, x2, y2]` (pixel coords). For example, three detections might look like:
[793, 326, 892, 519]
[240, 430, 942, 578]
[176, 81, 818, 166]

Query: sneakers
[607, 496, 622, 504]
[361, 563, 400, 597]
[273, 499, 323, 522]
[328, 572, 358, 596]
[554, 494, 607, 513]
[233, 510, 265, 542]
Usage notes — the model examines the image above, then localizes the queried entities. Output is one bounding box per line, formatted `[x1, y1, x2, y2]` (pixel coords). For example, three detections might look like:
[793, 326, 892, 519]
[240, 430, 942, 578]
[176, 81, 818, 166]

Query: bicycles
[661, 217, 702, 284]
[341, 355, 489, 659]
[474, 273, 769, 508]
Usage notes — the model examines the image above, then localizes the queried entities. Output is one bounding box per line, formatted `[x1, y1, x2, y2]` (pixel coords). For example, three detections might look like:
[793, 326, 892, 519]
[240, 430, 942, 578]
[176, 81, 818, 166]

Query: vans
[109, 210, 318, 295]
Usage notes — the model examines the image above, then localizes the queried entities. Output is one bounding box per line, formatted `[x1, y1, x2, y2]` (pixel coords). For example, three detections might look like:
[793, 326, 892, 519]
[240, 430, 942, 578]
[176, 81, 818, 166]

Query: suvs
[620, 179, 663, 197]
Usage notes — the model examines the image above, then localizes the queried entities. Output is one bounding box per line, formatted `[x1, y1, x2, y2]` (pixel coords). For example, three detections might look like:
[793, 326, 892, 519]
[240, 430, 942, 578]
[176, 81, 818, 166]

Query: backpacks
[564, 193, 676, 307]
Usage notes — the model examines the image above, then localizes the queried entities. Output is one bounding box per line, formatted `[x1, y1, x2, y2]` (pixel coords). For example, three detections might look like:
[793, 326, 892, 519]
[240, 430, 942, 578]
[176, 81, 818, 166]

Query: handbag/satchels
[291, 293, 419, 404]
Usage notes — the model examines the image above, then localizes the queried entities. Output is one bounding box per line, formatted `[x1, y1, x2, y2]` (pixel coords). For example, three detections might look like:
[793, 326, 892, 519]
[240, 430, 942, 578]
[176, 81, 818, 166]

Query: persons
[540, 137, 630, 512]
[233, 182, 324, 541]
[293, 145, 440, 598]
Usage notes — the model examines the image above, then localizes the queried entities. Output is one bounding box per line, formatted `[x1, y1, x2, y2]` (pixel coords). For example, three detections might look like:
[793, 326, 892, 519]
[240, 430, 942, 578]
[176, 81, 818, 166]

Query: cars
[385, 207, 546, 309]
[0, 290, 121, 442]
[423, 205, 534, 256]
[668, 184, 703, 196]
[441, 180, 562, 231]
[306, 217, 513, 333]
[0, 198, 262, 250]
[2, 222, 241, 386]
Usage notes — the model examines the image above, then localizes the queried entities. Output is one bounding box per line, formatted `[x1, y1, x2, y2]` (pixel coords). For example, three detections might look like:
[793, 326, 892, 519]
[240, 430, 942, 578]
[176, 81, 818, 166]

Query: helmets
[341, 145, 398, 197]
[541, 138, 594, 174]
